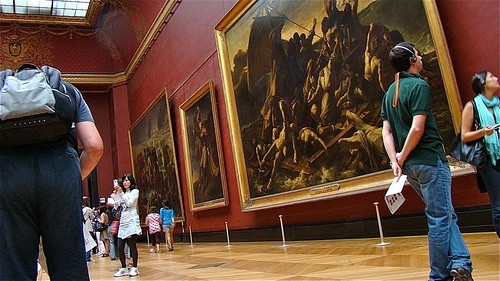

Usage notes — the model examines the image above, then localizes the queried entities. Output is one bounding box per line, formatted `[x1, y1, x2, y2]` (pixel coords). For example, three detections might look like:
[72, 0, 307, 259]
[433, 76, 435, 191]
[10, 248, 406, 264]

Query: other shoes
[449, 266, 473, 281]
[168, 246, 174, 250]
[87, 260, 95, 263]
[150, 249, 162, 253]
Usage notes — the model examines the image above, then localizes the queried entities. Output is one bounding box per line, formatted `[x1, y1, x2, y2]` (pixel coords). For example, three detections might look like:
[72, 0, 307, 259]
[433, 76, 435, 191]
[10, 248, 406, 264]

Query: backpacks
[0, 65, 76, 145]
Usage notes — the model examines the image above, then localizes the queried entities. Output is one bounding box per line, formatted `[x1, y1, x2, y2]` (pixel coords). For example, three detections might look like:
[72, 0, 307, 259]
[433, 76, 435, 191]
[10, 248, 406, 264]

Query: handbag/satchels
[96, 214, 108, 232]
[109, 207, 123, 235]
[459, 98, 489, 169]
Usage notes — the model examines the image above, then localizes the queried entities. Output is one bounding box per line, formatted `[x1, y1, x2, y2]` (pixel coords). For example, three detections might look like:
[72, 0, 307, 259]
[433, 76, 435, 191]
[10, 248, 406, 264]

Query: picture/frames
[128, 87, 185, 227]
[179, 81, 229, 213]
[213, 0, 476, 213]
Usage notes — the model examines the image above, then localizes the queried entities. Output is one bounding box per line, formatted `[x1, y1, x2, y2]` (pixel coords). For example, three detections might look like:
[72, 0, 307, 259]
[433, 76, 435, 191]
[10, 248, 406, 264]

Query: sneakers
[113, 268, 128, 276]
[128, 266, 140, 276]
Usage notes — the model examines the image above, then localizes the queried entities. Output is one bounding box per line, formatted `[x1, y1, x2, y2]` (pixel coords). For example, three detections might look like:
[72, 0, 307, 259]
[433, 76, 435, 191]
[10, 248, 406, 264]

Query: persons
[461, 71, 500, 238]
[0, 63, 104, 281]
[105, 210, 116, 260]
[82, 196, 95, 232]
[98, 206, 108, 257]
[83, 224, 97, 261]
[160, 200, 175, 251]
[113, 174, 143, 276]
[382, 42, 472, 281]
[145, 206, 162, 253]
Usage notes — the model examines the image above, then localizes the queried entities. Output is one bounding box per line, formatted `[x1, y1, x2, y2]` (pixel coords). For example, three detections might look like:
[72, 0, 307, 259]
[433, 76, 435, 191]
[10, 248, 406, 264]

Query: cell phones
[113, 179, 118, 187]
